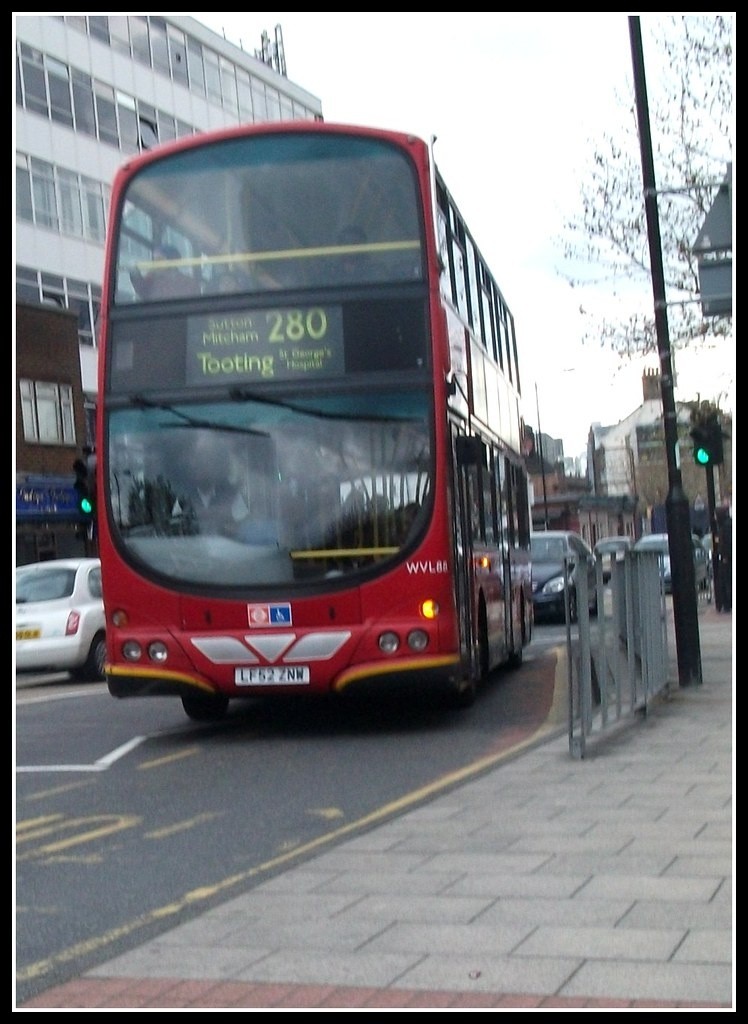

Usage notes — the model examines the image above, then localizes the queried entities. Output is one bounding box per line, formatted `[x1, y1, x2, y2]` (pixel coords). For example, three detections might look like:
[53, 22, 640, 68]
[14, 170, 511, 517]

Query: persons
[334, 224, 373, 285]
[341, 489, 421, 569]
[128, 245, 195, 301]
[170, 458, 249, 526]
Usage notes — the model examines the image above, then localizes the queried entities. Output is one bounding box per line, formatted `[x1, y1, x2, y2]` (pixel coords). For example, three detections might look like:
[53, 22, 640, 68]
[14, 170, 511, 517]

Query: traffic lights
[691, 420, 722, 469]
[75, 453, 96, 527]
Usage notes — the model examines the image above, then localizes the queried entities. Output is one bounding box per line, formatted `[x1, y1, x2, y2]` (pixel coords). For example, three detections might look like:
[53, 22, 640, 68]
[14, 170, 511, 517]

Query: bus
[92, 118, 533, 722]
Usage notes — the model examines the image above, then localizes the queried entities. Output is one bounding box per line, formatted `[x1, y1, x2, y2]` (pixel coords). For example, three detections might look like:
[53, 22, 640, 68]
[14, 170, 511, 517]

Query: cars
[592, 537, 632, 574]
[701, 532, 714, 574]
[16, 558, 105, 683]
[529, 530, 598, 622]
[635, 534, 708, 594]
